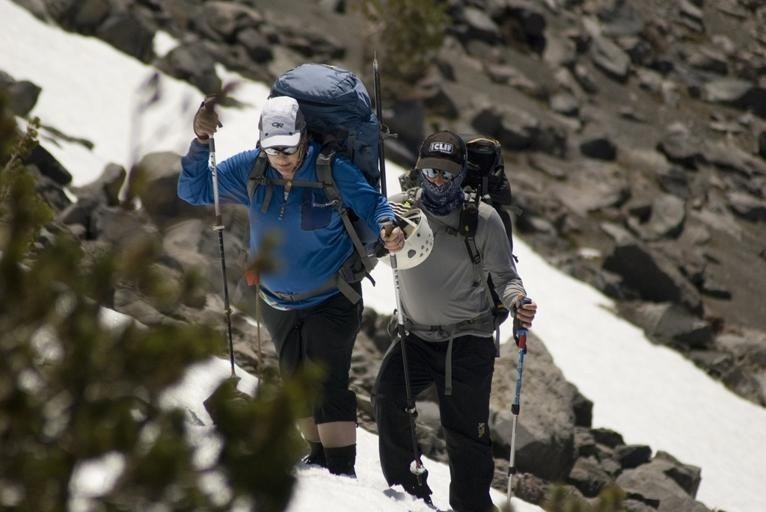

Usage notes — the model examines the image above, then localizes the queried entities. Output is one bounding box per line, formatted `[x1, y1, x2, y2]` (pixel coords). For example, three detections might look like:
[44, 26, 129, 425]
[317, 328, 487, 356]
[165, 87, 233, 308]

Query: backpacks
[245, 63, 385, 282]
[398, 137, 515, 335]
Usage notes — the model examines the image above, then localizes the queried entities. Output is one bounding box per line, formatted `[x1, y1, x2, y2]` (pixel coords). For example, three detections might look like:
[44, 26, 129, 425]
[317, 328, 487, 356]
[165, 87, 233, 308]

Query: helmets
[383, 210, 434, 270]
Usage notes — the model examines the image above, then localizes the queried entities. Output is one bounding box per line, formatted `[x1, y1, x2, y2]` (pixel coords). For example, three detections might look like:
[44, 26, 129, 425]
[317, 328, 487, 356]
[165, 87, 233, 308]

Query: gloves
[193, 95, 223, 146]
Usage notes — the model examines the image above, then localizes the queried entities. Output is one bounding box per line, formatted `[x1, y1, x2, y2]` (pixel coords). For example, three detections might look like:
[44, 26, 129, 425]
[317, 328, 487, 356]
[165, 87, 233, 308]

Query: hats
[415, 130, 465, 177]
[257, 95, 307, 150]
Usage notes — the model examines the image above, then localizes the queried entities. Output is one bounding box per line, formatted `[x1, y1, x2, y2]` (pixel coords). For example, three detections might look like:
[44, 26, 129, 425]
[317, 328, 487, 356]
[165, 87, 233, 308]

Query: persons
[371, 131, 538, 512]
[177, 93, 407, 478]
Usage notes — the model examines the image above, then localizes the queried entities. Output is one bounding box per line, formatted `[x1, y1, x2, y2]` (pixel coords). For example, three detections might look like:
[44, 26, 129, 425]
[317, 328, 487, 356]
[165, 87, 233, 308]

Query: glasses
[420, 167, 458, 181]
[261, 147, 300, 156]
